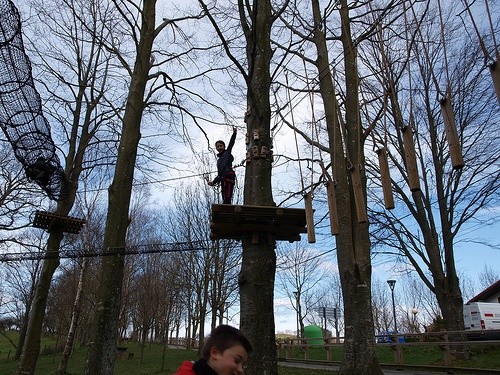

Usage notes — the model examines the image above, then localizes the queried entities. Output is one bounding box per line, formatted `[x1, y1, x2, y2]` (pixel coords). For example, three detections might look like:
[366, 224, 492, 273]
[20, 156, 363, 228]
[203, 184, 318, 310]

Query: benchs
[128, 353, 134, 360]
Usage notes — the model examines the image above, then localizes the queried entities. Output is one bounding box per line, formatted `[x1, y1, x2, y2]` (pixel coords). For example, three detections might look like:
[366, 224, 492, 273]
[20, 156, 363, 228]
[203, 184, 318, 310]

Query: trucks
[462, 302, 500, 341]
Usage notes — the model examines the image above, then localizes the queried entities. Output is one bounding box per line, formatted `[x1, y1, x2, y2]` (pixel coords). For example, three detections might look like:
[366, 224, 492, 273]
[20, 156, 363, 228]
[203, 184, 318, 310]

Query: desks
[117, 347, 129, 359]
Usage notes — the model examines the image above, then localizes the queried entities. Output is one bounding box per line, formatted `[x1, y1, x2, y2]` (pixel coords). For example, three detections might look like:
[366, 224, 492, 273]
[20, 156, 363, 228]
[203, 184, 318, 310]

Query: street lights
[293, 291, 299, 344]
[387, 279, 399, 342]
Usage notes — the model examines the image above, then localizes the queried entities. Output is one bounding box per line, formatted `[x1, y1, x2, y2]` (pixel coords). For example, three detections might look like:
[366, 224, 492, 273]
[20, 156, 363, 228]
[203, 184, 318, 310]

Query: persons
[423, 325, 427, 333]
[383, 331, 386, 343]
[171, 325, 253, 375]
[207, 127, 237, 204]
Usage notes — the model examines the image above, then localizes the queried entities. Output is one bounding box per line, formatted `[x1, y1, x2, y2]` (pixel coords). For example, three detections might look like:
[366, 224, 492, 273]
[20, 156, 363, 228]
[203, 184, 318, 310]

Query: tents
[304, 324, 325, 344]
